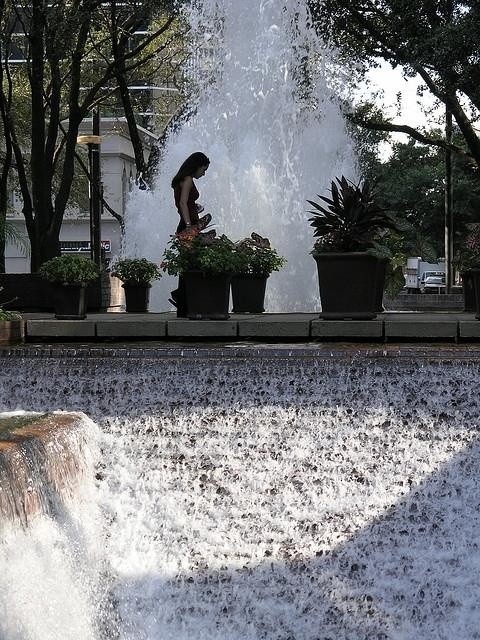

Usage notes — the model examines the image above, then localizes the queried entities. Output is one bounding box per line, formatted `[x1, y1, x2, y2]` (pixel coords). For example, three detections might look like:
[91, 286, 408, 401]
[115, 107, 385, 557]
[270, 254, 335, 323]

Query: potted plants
[36, 230, 289, 321]
[306, 172, 408, 319]
[451, 220, 479, 319]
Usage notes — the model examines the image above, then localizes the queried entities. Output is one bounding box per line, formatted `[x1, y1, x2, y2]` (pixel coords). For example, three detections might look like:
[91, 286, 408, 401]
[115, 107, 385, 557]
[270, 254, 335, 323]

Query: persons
[166, 151, 210, 307]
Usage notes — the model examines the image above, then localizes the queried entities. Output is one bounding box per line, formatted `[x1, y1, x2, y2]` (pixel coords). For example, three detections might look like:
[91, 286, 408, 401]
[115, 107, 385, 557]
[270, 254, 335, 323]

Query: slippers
[168, 298, 177, 308]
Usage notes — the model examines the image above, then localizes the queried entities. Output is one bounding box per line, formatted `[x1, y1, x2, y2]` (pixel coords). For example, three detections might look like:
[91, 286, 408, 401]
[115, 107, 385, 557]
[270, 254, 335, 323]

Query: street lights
[77, 135, 103, 277]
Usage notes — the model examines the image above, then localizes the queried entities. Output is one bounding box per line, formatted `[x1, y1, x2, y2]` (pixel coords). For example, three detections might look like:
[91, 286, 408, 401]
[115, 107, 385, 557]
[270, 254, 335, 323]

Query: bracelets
[185, 223, 192, 226]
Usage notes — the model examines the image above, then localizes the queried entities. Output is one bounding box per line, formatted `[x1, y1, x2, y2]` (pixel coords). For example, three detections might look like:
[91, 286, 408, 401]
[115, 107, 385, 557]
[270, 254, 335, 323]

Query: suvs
[419, 271, 446, 293]
